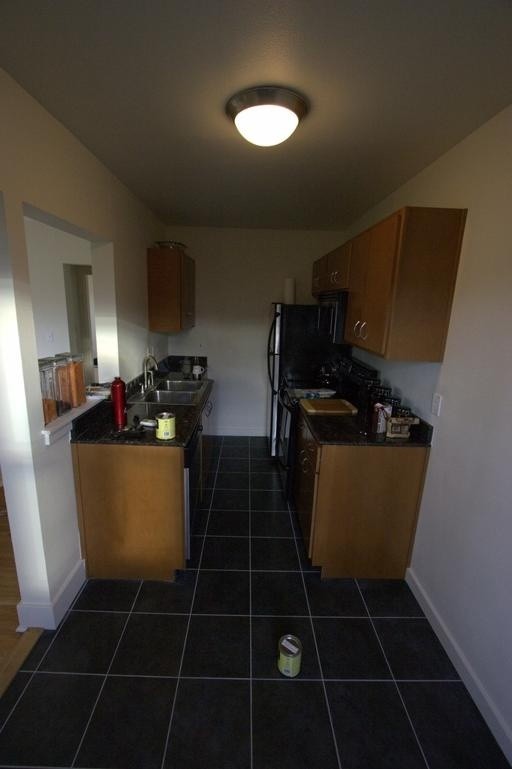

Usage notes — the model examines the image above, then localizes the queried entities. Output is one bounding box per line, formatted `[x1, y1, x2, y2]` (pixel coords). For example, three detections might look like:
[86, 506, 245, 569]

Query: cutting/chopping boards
[299, 397, 358, 415]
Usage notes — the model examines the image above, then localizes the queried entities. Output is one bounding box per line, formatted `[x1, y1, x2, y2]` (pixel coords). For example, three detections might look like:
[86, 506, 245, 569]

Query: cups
[193, 366, 205, 374]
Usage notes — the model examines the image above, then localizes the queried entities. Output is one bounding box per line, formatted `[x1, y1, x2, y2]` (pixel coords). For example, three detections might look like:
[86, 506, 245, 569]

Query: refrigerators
[266, 302, 336, 458]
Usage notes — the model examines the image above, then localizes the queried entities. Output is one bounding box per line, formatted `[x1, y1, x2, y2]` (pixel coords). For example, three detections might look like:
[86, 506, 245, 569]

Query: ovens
[274, 391, 299, 498]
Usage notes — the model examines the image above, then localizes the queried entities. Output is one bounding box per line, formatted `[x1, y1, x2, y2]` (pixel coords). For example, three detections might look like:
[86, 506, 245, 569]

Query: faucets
[144, 355, 159, 388]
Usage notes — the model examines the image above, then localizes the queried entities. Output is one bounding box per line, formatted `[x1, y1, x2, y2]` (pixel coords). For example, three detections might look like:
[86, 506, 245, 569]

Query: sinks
[156, 380, 209, 391]
[143, 390, 201, 405]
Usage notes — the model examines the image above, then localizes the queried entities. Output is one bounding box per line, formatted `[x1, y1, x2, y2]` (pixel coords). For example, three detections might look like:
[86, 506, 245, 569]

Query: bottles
[112, 377, 127, 425]
[39, 351, 87, 426]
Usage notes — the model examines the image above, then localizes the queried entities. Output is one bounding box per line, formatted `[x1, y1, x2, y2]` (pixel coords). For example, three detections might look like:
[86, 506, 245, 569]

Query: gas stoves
[283, 375, 344, 405]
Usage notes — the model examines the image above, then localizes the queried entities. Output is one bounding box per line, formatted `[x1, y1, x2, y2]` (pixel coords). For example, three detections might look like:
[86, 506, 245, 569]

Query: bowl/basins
[154, 239, 188, 251]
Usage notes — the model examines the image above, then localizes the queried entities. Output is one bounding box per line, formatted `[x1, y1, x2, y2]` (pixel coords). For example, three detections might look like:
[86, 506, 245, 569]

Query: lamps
[224, 85, 309, 149]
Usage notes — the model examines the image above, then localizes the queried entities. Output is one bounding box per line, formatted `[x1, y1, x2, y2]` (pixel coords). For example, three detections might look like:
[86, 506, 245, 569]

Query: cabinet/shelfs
[291, 412, 432, 580]
[146, 245, 195, 333]
[200, 382, 220, 493]
[309, 205, 467, 365]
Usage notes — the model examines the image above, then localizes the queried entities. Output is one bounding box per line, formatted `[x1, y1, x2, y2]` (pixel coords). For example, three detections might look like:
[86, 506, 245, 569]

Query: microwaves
[314, 291, 347, 345]
